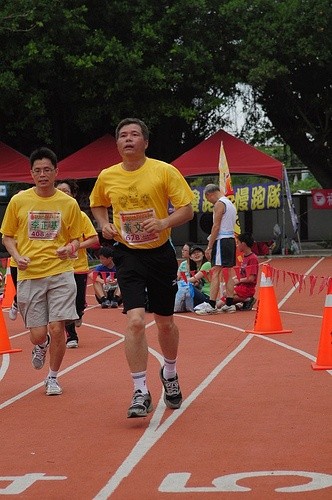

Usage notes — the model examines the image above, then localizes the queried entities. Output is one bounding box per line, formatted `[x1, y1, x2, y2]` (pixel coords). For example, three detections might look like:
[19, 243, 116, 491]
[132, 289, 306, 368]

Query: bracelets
[68, 244, 74, 255]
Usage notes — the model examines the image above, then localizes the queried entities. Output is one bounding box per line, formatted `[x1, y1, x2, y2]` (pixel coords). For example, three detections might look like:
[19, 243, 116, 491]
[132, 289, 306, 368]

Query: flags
[218, 146, 241, 236]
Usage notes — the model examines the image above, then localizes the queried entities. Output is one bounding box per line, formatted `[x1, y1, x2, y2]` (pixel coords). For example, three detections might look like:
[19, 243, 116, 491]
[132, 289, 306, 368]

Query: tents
[0, 134, 122, 185]
[169, 129, 305, 255]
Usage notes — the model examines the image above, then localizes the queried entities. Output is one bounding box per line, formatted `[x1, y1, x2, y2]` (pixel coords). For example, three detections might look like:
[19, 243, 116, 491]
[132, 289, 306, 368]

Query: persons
[88, 117, 195, 418]
[56, 181, 101, 349]
[195, 183, 237, 315]
[88, 249, 121, 308]
[176, 242, 211, 301]
[217, 232, 258, 311]
[0, 146, 84, 395]
[8, 189, 26, 321]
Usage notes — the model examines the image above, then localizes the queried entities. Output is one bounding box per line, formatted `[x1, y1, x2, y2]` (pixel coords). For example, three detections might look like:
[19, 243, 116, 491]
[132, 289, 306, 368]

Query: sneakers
[101, 300, 110, 309]
[111, 300, 118, 308]
[195, 302, 217, 315]
[8, 303, 18, 320]
[75, 318, 83, 327]
[218, 305, 237, 314]
[244, 297, 256, 311]
[31, 334, 51, 369]
[44, 376, 63, 396]
[159, 366, 183, 410]
[127, 389, 153, 418]
[66, 340, 79, 348]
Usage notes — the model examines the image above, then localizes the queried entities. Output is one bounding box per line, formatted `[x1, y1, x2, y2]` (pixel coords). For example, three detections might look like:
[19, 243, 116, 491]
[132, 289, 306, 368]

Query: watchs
[207, 247, 212, 250]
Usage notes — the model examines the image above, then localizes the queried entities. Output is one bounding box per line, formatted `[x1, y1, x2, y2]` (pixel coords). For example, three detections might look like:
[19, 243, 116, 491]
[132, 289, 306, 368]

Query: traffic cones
[311, 276, 332, 370]
[0, 304, 24, 355]
[244, 257, 294, 335]
[0, 258, 17, 309]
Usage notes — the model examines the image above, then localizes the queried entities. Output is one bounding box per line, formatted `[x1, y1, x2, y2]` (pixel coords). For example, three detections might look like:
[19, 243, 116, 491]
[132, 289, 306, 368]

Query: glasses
[33, 168, 55, 173]
[182, 249, 188, 252]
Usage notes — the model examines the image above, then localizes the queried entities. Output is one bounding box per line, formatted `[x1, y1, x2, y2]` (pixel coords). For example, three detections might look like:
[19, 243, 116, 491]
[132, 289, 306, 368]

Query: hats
[189, 245, 204, 254]
